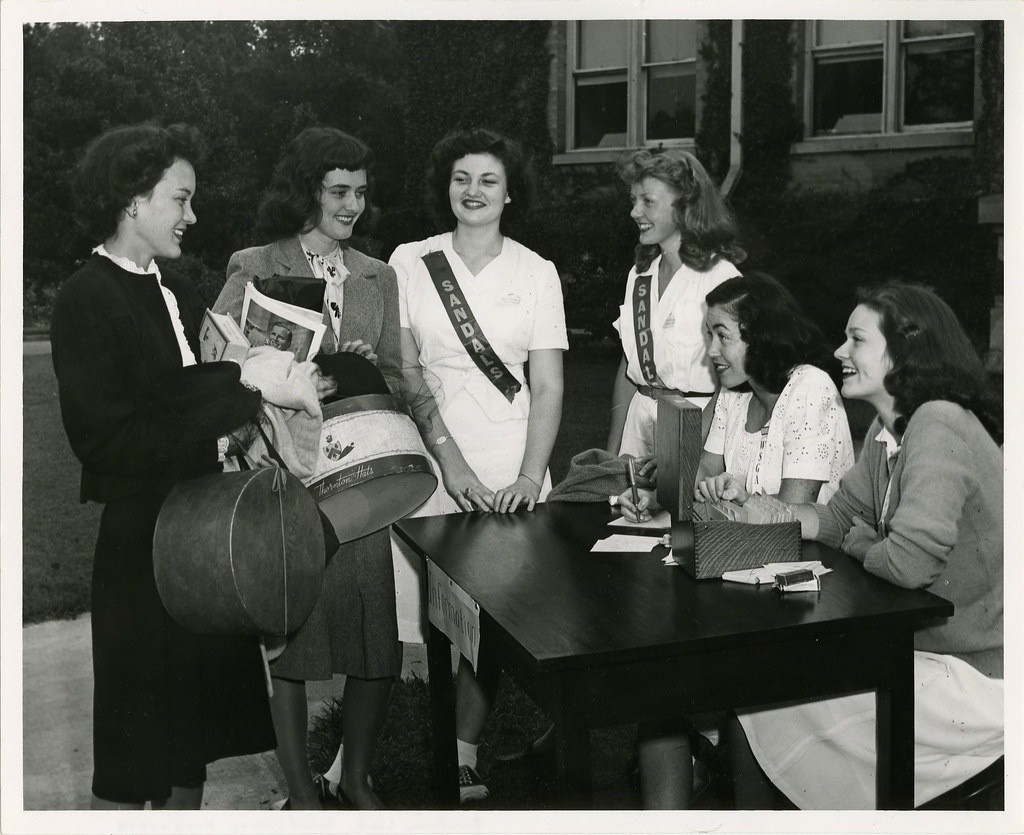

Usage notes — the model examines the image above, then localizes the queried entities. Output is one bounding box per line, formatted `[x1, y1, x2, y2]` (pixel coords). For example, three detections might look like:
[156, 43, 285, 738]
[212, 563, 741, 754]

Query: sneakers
[459, 765, 489, 801]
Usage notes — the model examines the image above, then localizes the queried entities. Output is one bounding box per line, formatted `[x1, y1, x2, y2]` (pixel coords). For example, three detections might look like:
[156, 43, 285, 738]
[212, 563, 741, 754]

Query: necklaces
[660, 233, 680, 256]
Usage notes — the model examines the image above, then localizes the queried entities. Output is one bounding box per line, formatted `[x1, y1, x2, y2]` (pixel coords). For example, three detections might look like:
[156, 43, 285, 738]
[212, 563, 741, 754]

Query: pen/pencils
[628, 458, 641, 523]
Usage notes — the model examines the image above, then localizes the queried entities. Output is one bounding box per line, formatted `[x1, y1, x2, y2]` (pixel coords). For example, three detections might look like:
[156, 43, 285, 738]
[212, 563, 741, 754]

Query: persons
[204, 122, 409, 811]
[266, 123, 572, 811]
[268, 321, 293, 352]
[692, 278, 1002, 811]
[44, 118, 325, 810]
[603, 147, 746, 507]
[616, 270, 856, 810]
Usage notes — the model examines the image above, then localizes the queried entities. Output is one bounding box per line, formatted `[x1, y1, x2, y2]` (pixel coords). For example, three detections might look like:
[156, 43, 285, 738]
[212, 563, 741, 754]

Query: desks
[385, 503, 956, 810]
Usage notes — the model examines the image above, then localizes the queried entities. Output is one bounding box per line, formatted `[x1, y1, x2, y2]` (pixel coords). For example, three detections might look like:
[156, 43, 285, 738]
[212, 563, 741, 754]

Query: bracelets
[427, 433, 453, 452]
[517, 472, 543, 492]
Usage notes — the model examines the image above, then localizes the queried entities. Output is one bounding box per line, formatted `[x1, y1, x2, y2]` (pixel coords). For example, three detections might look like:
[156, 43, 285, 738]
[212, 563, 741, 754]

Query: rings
[463, 487, 473, 496]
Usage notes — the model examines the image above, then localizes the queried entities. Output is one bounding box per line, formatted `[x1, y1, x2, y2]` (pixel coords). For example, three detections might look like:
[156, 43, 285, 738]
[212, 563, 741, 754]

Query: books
[195, 308, 251, 364]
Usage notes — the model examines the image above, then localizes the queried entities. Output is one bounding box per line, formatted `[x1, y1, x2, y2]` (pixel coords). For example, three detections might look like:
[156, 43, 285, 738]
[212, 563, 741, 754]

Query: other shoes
[337, 785, 384, 809]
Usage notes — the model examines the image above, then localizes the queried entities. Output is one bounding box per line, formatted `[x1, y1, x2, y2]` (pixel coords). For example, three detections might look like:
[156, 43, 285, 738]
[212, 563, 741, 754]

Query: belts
[637, 384, 714, 400]
